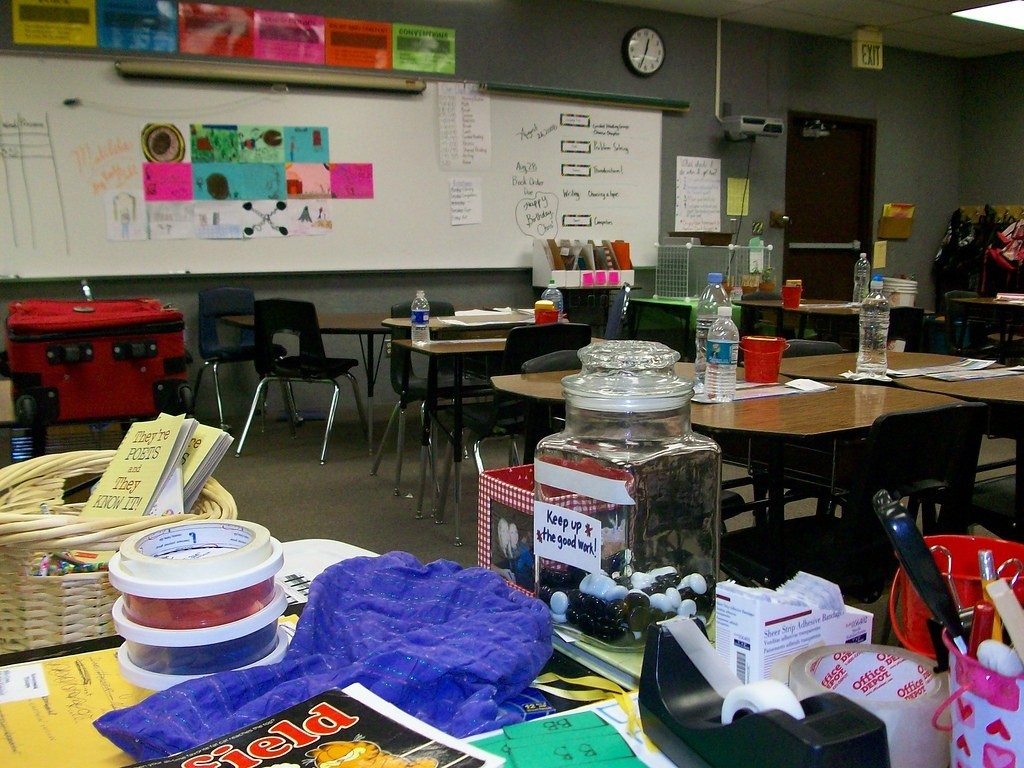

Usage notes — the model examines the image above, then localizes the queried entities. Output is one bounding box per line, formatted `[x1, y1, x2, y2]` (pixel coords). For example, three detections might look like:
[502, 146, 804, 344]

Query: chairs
[737, 292, 782, 336]
[783, 336, 844, 360]
[624, 298, 692, 356]
[191, 283, 306, 431]
[369, 300, 494, 498]
[232, 297, 372, 466]
[427, 323, 592, 523]
[516, 340, 746, 536]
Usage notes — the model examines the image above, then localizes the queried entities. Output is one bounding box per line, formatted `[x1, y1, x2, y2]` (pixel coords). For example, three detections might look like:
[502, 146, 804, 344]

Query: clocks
[617, 25, 668, 77]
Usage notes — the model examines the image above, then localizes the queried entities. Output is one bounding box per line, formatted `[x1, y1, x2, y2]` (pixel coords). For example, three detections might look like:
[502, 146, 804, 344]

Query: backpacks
[930, 203, 1024, 319]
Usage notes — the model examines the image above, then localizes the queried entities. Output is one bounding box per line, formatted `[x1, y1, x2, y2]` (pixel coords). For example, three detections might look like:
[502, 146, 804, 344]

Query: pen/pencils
[965, 600, 995, 661]
[977, 549, 1003, 643]
[985, 579, 1023, 665]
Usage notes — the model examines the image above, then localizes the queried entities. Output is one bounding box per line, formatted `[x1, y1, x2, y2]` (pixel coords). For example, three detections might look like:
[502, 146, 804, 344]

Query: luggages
[5, 296, 194, 426]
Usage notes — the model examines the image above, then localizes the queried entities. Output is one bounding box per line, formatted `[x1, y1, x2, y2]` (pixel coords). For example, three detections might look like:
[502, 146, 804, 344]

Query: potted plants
[741, 286, 757, 294]
[758, 264, 778, 292]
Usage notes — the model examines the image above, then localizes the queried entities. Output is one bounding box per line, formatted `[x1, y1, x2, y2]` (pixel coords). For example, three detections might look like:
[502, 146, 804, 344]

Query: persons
[490, 518, 535, 593]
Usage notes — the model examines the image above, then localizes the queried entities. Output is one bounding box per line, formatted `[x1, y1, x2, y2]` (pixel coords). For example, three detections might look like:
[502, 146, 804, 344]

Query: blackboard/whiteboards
[0, 43, 664, 287]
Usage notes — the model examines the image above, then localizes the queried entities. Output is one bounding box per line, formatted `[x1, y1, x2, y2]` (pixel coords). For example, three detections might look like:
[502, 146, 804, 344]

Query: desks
[223, 297, 1024, 587]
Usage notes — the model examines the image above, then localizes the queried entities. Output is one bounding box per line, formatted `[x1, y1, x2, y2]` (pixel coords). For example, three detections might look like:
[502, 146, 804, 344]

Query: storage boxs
[472, 463, 635, 606]
[531, 238, 636, 288]
[715, 582, 877, 687]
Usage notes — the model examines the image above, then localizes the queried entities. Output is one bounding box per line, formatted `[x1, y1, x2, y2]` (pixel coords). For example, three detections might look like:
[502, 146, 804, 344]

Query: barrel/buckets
[882, 277, 918, 309]
[739, 334, 790, 384]
[781, 286, 803, 310]
[889, 534, 1024, 660]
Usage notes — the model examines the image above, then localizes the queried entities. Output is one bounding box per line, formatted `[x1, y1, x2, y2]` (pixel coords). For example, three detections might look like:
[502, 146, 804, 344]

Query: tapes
[784, 642, 954, 768]
[121, 517, 270, 582]
[663, 617, 805, 725]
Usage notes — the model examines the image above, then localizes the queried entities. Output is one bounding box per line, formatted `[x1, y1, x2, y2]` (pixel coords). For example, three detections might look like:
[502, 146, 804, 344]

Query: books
[63, 411, 235, 563]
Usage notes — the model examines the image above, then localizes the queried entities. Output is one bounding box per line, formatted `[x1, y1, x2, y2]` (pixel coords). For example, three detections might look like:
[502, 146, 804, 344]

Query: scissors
[871, 489, 967, 655]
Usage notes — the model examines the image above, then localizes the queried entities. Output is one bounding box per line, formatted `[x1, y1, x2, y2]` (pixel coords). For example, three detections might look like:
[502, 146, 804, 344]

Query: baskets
[0, 449, 238, 655]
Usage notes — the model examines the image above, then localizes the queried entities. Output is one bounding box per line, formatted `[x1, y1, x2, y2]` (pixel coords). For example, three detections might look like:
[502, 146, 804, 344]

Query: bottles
[852, 253, 870, 307]
[541, 280, 563, 323]
[704, 308, 739, 402]
[694, 273, 732, 384]
[856, 280, 891, 378]
[532, 338, 724, 655]
[411, 290, 431, 347]
